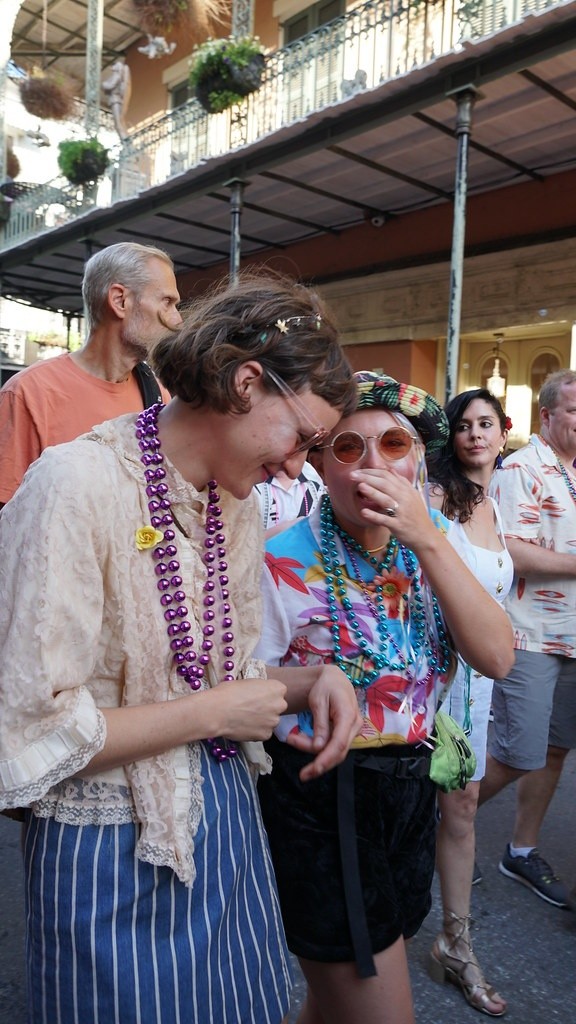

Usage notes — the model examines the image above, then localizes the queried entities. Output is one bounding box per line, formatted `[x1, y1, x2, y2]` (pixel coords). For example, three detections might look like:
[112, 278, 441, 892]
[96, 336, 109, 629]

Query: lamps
[486, 333, 506, 397]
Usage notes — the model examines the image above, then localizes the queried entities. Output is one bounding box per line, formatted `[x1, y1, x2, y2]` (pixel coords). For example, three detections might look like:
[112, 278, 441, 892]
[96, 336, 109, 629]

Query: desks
[0, 181, 67, 226]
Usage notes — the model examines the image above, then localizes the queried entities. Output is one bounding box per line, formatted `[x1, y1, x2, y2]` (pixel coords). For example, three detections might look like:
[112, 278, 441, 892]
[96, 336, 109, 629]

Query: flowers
[188, 31, 277, 112]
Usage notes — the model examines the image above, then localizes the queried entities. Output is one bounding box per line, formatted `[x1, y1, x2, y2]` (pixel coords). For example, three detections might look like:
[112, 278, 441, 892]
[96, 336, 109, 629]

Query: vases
[196, 54, 265, 114]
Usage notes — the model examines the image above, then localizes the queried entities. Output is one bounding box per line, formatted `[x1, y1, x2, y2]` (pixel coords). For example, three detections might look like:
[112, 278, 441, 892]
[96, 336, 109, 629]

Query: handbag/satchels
[429, 711, 477, 794]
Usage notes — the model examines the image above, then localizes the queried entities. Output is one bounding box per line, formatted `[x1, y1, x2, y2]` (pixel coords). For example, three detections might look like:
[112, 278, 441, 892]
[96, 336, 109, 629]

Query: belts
[337, 755, 432, 977]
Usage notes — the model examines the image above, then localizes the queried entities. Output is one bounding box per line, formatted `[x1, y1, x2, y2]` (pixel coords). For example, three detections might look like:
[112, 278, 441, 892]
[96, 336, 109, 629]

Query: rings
[387, 501, 399, 516]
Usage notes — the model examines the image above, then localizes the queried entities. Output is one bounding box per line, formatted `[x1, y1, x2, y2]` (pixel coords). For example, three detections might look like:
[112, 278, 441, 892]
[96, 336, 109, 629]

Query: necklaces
[320, 494, 451, 688]
[540, 434, 576, 503]
[271, 483, 309, 524]
[136, 403, 240, 762]
[368, 544, 387, 553]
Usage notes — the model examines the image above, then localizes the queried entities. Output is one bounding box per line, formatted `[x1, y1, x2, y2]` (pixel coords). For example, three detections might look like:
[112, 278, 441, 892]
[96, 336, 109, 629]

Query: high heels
[430, 907, 508, 1016]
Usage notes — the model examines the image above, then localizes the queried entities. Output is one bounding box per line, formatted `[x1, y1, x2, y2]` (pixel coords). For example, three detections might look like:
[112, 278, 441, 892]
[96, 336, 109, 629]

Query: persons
[254, 470, 323, 542]
[0, 241, 186, 506]
[472, 367, 576, 905]
[0, 270, 361, 1024]
[422, 389, 515, 1016]
[263, 371, 516, 1024]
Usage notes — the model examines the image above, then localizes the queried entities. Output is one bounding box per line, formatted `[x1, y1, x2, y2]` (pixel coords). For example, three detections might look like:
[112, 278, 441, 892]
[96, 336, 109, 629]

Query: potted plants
[56, 134, 112, 187]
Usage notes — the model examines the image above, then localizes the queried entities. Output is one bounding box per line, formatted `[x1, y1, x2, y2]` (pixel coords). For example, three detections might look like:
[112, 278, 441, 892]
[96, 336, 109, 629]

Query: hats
[345, 371, 450, 456]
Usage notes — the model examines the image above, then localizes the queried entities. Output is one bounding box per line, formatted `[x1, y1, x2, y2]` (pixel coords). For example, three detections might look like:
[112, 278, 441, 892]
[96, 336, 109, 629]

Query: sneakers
[471, 863, 482, 885]
[499, 842, 570, 908]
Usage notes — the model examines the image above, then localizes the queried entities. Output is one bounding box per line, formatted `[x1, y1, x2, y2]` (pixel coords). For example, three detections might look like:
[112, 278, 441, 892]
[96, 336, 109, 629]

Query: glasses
[260, 364, 330, 459]
[317, 427, 419, 465]
